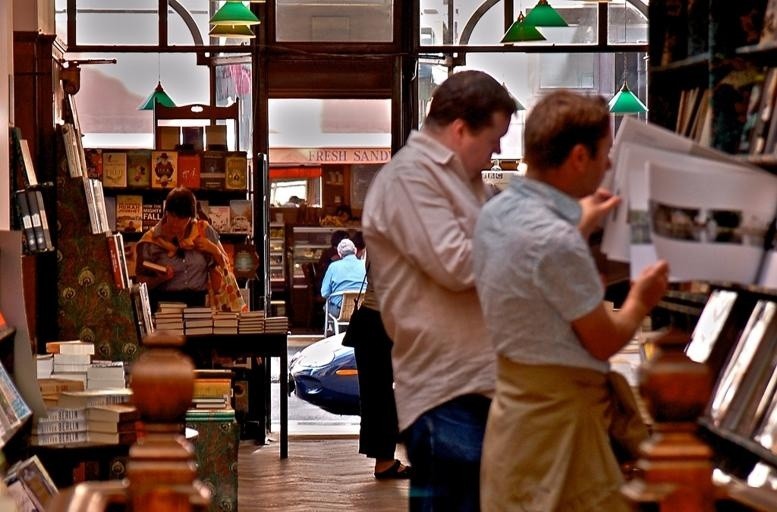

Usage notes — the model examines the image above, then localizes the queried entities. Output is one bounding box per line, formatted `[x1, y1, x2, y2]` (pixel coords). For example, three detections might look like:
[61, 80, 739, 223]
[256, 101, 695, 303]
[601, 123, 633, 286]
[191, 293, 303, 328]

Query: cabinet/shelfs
[268, 223, 364, 327]
[0, 96, 269, 512]
[13, 30, 68, 356]
[646, 3, 775, 511]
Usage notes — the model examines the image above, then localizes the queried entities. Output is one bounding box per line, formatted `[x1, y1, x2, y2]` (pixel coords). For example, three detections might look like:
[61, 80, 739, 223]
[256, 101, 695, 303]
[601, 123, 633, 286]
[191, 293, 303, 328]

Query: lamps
[209, 1, 262, 40]
[136, 51, 176, 111]
[500, 0, 569, 45]
[609, 0, 649, 114]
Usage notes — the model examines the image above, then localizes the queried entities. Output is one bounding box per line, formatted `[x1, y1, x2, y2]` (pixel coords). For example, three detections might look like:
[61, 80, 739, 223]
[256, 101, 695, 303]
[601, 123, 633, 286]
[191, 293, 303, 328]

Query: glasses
[170, 235, 186, 259]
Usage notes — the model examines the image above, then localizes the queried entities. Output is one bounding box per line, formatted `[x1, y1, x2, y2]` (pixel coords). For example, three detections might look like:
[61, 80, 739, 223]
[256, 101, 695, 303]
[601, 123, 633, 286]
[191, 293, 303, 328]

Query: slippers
[374, 459, 411, 479]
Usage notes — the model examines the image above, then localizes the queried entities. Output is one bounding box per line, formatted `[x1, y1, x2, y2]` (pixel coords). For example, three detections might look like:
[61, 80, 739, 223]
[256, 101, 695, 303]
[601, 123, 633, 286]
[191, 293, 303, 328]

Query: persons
[362, 69, 516, 512]
[316, 205, 367, 332]
[310, 280, 311, 281]
[351, 252, 412, 482]
[472, 86, 672, 512]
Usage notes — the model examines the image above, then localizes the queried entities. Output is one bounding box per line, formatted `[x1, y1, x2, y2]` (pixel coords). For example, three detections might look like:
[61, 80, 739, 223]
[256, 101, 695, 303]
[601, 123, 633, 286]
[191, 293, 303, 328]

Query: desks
[152, 332, 288, 460]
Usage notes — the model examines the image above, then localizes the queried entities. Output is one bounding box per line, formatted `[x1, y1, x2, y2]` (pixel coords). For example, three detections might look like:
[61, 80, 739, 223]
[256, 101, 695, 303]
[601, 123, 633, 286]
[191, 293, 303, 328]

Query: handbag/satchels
[342, 305, 394, 347]
